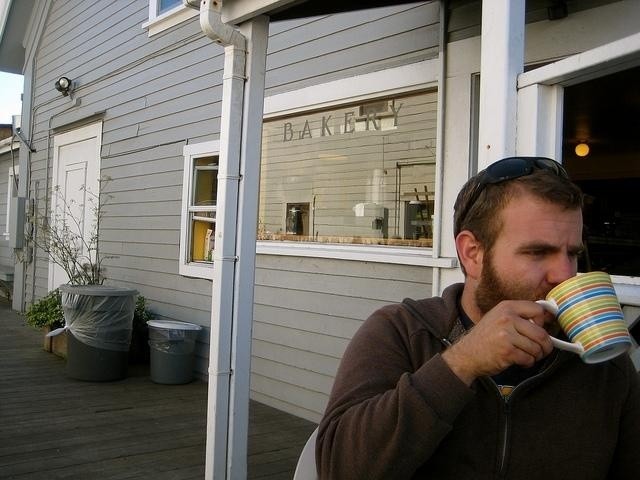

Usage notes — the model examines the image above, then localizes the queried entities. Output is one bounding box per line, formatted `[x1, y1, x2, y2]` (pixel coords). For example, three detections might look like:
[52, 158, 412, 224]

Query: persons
[314, 158, 640, 480]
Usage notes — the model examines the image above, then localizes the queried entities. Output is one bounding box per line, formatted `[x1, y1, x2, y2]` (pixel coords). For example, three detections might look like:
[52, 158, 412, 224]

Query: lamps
[55, 76, 76, 100]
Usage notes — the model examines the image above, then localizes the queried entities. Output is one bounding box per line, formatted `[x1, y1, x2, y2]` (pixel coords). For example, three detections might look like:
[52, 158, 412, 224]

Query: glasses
[455, 156, 570, 231]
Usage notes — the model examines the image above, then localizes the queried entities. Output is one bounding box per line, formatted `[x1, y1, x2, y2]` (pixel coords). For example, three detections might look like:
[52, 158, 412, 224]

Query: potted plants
[15, 287, 69, 359]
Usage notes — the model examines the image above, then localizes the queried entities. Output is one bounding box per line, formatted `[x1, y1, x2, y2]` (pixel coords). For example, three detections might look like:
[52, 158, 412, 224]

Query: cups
[528, 271, 633, 365]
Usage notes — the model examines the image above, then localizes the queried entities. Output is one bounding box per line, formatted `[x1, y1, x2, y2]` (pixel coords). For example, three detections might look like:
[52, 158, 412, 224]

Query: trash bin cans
[147, 320, 202, 385]
[61, 284, 139, 382]
